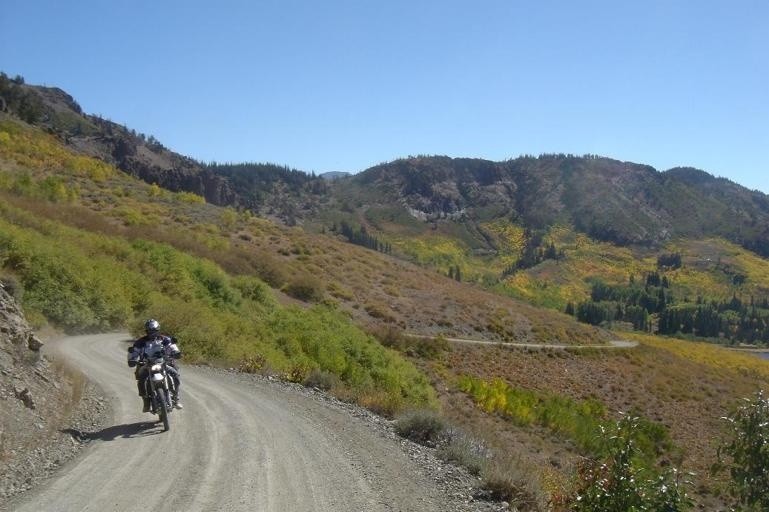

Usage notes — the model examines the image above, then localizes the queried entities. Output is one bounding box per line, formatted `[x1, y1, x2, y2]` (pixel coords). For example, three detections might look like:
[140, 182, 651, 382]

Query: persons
[126, 318, 183, 413]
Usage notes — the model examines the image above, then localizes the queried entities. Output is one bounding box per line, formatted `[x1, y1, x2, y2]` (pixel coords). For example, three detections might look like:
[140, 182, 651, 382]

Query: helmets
[144, 319, 160, 338]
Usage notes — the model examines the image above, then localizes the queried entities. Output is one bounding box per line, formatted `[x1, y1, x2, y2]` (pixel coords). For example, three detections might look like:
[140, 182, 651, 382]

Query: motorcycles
[124, 336, 183, 431]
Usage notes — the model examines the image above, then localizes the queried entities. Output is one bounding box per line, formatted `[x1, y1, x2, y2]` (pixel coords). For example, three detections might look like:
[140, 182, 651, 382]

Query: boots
[171, 395, 183, 408]
[142, 398, 150, 412]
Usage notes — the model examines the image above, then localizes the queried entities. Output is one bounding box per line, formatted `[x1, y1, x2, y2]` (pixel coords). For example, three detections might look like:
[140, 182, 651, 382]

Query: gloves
[128, 359, 136, 366]
[171, 351, 181, 358]
